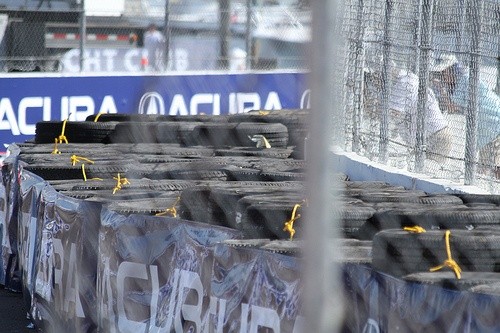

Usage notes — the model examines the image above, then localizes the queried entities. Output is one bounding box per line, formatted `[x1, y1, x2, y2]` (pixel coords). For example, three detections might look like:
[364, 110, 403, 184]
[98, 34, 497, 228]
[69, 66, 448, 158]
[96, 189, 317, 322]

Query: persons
[143, 25, 163, 68]
[389, 60, 453, 167]
[430, 55, 500, 175]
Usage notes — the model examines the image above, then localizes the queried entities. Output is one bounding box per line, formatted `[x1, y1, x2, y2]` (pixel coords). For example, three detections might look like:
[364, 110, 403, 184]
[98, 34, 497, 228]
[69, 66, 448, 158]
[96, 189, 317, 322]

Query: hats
[364, 55, 407, 79]
[431, 55, 457, 71]
